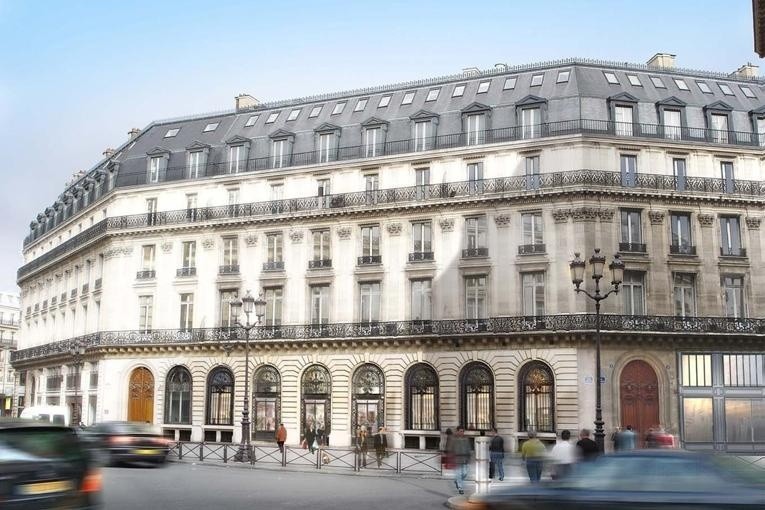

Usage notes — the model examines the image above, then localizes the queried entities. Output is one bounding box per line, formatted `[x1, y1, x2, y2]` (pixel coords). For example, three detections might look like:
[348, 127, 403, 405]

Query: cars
[78, 420, 170, 470]
[468, 448, 765, 508]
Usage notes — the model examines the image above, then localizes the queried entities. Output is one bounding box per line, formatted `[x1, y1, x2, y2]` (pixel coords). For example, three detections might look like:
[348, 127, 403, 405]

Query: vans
[18, 405, 72, 427]
[0, 415, 104, 509]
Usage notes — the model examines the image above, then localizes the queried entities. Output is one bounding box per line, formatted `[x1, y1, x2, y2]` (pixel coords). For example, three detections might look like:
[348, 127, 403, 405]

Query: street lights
[70, 337, 85, 427]
[230, 286, 268, 463]
[567, 244, 625, 452]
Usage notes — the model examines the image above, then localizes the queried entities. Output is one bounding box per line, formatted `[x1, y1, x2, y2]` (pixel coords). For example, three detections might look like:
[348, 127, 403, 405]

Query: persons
[353, 415, 387, 471]
[576, 428, 600, 465]
[549, 429, 576, 481]
[304, 415, 325, 452]
[488, 426, 506, 482]
[611, 424, 665, 452]
[522, 429, 547, 483]
[275, 423, 287, 454]
[442, 428, 455, 469]
[448, 426, 471, 494]
